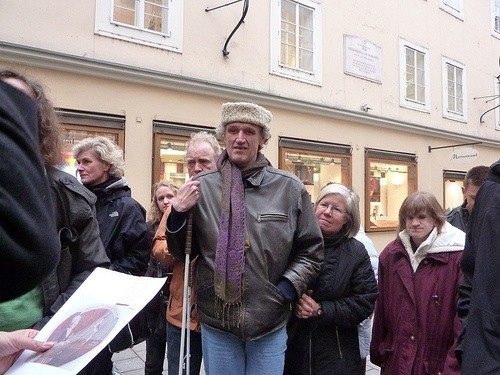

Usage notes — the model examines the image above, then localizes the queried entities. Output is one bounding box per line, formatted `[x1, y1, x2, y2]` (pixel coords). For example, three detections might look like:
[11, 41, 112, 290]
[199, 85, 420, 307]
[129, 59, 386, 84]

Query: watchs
[317, 304, 323, 316]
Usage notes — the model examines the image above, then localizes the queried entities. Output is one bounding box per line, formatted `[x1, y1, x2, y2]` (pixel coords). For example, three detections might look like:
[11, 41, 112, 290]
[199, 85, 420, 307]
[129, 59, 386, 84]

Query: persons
[0, 70, 500, 375]
[38, 314, 99, 365]
[164, 102, 325, 375]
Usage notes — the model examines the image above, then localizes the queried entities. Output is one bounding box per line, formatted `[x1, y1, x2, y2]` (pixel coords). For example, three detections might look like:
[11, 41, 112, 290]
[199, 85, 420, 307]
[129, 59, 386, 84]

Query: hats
[216, 101, 272, 146]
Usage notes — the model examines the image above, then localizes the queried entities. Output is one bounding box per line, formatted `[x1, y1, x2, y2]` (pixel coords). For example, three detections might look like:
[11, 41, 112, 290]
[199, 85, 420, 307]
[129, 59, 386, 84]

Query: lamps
[380, 172, 387, 185]
